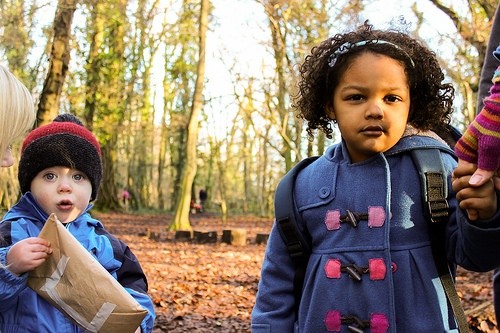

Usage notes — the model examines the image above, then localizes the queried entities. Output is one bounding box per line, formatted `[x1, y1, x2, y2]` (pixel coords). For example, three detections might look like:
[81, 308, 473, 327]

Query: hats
[18, 114, 103, 202]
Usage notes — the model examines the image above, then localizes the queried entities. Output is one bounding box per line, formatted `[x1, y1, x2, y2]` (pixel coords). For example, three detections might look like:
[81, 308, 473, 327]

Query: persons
[121, 186, 129, 205]
[0, 112, 157, 333]
[454, 43, 500, 220]
[190, 187, 208, 213]
[250, 19, 500, 332]
[0, 65, 35, 168]
